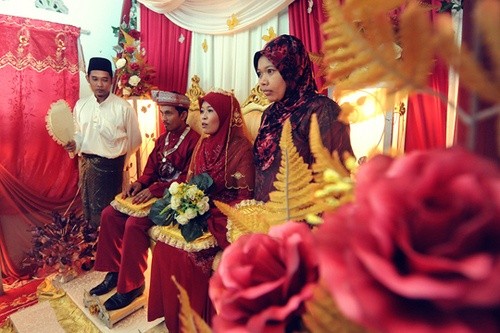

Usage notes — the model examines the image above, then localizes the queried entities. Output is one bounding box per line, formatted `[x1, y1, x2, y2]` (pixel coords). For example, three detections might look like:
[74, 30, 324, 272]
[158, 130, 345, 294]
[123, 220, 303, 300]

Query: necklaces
[162, 127, 190, 163]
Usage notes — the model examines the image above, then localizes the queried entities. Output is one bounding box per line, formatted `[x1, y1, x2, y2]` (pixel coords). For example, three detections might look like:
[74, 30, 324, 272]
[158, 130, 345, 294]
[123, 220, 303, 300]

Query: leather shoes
[104, 281, 145, 311]
[90, 271, 118, 296]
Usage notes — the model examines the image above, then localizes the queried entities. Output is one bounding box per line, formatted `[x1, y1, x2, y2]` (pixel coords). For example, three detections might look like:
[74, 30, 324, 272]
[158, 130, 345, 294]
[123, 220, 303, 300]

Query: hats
[151, 90, 191, 109]
[88, 56, 113, 84]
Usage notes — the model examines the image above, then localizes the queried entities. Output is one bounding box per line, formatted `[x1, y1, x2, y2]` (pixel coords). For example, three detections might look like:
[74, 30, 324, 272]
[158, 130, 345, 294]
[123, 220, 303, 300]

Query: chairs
[241, 82, 274, 146]
[183, 76, 211, 140]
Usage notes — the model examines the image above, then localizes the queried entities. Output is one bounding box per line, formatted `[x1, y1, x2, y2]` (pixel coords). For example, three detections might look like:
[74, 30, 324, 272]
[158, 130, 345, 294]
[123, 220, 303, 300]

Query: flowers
[25, 214, 102, 272]
[110, 7, 149, 98]
[207, 145, 500, 333]
[164, 180, 208, 226]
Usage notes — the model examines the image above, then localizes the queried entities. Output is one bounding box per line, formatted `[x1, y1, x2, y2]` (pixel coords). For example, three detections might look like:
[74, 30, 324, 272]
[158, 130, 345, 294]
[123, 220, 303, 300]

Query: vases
[52, 261, 79, 290]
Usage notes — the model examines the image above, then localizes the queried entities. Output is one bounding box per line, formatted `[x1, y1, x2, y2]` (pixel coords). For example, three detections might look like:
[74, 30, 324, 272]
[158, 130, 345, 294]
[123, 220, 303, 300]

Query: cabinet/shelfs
[123, 97, 160, 183]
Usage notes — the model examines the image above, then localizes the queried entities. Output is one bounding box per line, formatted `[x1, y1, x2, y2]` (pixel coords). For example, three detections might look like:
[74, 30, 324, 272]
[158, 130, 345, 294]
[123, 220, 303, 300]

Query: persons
[148, 90, 254, 333]
[253, 34, 356, 230]
[89, 90, 202, 311]
[72, 57, 142, 271]
